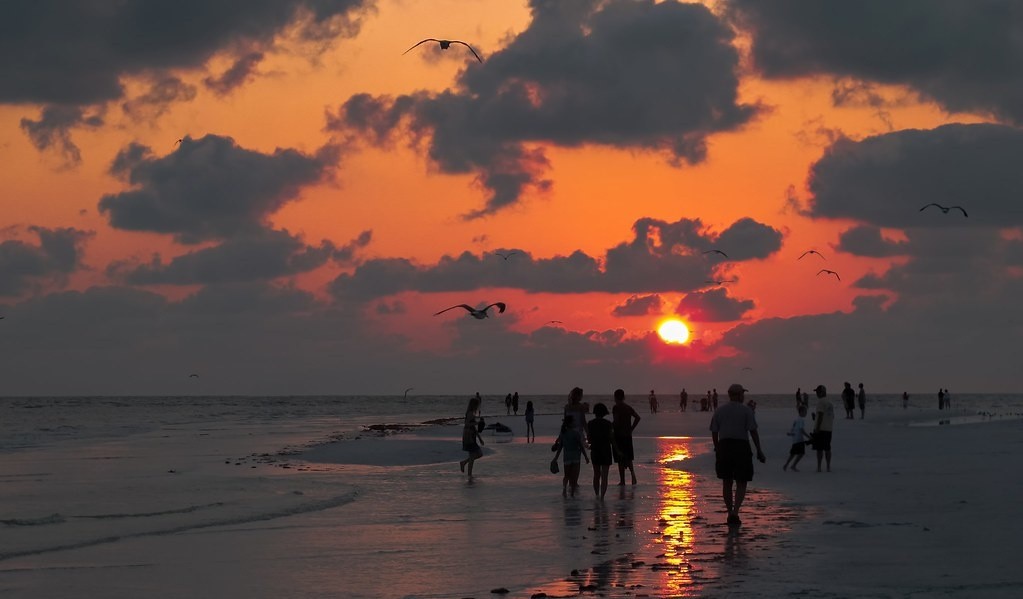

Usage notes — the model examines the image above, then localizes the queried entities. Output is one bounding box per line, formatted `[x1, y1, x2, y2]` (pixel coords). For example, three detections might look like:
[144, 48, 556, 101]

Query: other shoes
[551, 461, 559, 474]
[551, 443, 560, 452]
[727, 515, 742, 525]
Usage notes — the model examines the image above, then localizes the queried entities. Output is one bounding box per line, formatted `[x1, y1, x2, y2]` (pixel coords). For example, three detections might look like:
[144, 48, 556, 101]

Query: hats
[729, 384, 748, 394]
[814, 385, 827, 392]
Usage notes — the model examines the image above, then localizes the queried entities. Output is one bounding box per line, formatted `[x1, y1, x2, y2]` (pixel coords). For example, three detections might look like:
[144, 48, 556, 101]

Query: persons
[680, 388, 688, 412]
[748, 399, 756, 410]
[648, 390, 657, 414]
[938, 388, 950, 410]
[504, 391, 519, 415]
[783, 405, 810, 472]
[525, 401, 534, 437]
[812, 385, 834, 472]
[903, 392, 908, 407]
[858, 383, 866, 419]
[710, 383, 765, 523]
[460, 398, 484, 477]
[554, 386, 641, 498]
[474, 392, 482, 416]
[795, 388, 809, 407]
[707, 389, 718, 411]
[841, 382, 855, 418]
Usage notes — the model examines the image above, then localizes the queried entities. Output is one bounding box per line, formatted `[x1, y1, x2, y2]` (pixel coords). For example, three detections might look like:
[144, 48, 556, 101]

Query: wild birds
[704, 250, 728, 257]
[433, 302, 506, 320]
[705, 281, 734, 285]
[797, 250, 825, 259]
[816, 269, 840, 281]
[402, 39, 482, 63]
[919, 203, 968, 217]
